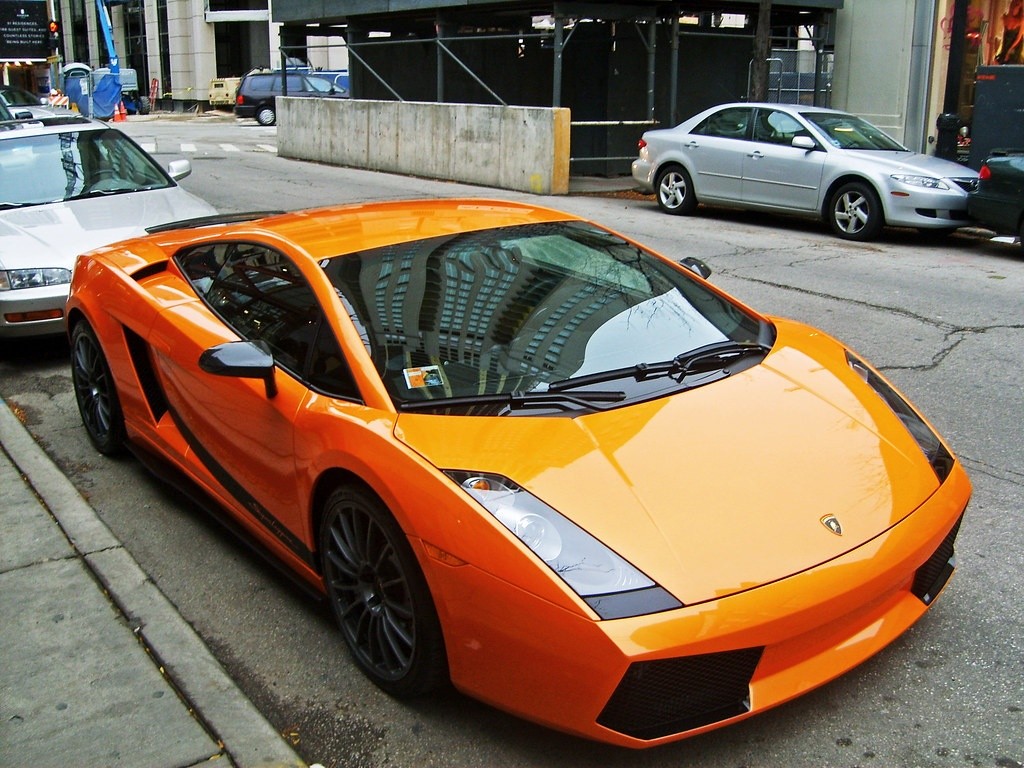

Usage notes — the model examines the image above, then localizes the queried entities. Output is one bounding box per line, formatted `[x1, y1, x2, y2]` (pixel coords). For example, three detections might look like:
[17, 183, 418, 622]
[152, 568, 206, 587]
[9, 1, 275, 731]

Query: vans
[208, 78, 240, 107]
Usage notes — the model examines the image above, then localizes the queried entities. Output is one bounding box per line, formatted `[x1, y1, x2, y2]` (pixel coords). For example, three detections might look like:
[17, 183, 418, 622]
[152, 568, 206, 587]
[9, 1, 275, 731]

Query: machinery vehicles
[62, 0, 150, 115]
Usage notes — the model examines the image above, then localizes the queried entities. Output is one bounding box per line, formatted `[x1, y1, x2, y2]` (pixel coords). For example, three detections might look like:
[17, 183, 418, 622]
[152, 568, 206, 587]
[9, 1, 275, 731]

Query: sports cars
[62, 196, 973, 751]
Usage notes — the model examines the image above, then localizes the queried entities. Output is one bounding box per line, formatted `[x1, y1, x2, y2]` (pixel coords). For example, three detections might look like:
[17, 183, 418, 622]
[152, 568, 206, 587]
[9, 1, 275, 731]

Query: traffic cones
[120, 102, 127, 121]
[112, 104, 121, 122]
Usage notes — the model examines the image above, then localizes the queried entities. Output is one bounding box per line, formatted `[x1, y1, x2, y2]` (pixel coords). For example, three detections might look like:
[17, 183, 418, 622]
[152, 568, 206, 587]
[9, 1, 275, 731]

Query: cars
[631, 98, 982, 241]
[234, 73, 349, 126]
[1, 99, 73, 177]
[969, 150, 1024, 242]
[0, 85, 82, 128]
[0, 116, 229, 340]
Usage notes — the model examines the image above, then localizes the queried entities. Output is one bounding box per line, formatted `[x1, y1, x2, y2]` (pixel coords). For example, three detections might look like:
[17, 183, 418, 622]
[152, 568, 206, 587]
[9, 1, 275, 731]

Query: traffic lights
[49, 20, 60, 40]
